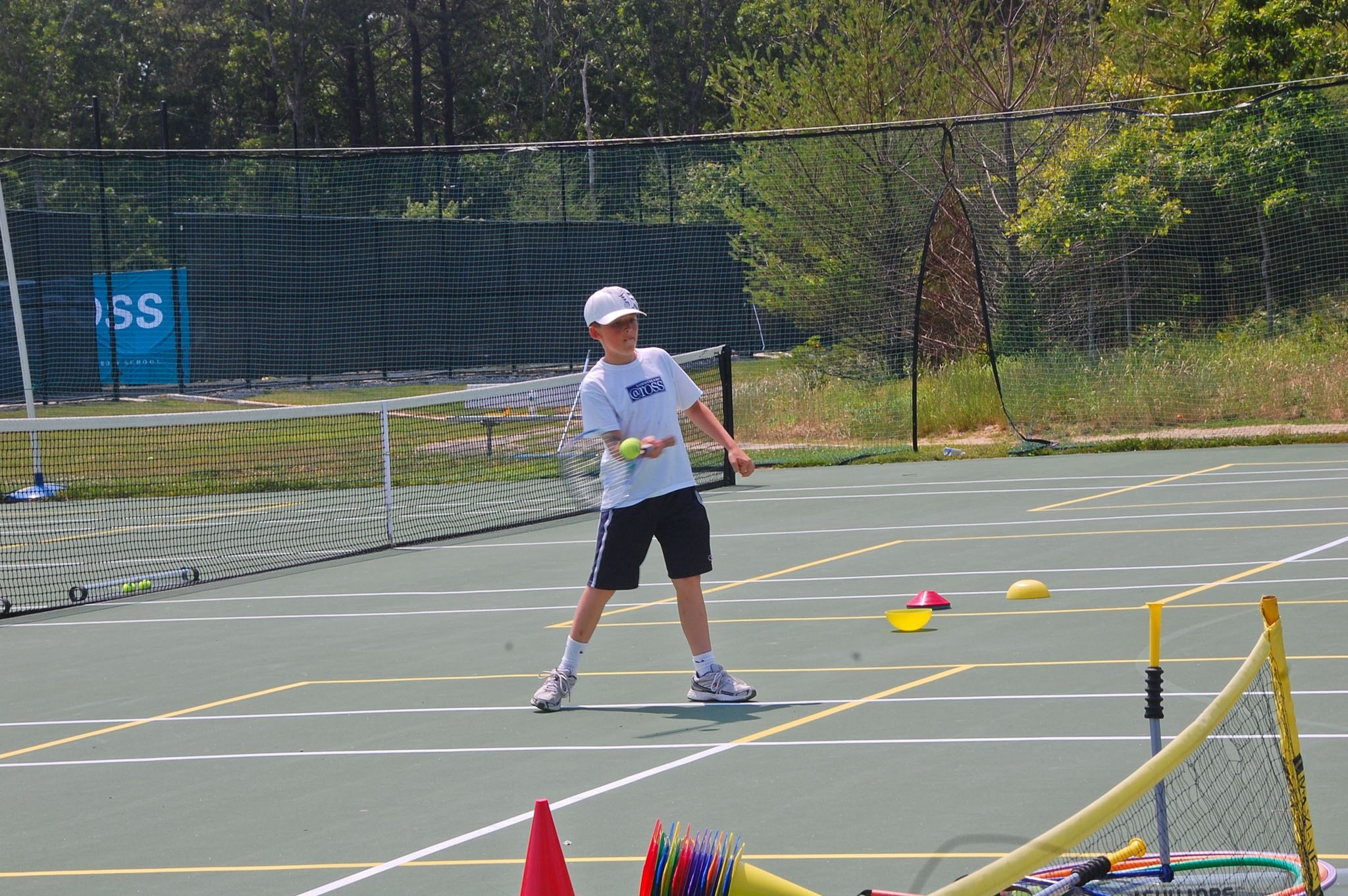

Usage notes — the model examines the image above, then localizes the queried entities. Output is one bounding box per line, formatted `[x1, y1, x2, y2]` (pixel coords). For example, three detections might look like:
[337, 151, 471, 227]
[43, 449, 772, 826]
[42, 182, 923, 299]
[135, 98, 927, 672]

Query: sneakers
[687, 666, 756, 701]
[530, 668, 578, 711]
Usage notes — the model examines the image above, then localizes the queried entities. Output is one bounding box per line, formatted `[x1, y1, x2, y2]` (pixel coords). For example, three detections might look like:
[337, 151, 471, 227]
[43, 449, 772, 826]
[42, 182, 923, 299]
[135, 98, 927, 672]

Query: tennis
[620, 438, 641, 460]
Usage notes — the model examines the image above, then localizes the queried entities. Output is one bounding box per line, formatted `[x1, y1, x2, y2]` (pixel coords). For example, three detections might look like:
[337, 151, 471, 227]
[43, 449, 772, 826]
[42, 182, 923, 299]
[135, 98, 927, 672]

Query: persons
[530, 285, 758, 711]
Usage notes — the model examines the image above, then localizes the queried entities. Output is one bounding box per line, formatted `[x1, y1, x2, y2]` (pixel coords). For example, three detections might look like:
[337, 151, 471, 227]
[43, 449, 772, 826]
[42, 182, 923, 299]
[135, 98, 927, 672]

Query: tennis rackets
[559, 428, 677, 514]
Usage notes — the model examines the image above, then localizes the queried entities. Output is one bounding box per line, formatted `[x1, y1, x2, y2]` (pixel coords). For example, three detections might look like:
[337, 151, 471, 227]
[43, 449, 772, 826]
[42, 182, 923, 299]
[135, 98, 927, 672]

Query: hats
[584, 285, 647, 327]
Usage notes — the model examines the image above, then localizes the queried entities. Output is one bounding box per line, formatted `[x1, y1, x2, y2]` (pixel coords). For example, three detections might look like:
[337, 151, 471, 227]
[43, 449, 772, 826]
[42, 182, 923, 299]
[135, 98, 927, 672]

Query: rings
[745, 470, 748, 472]
